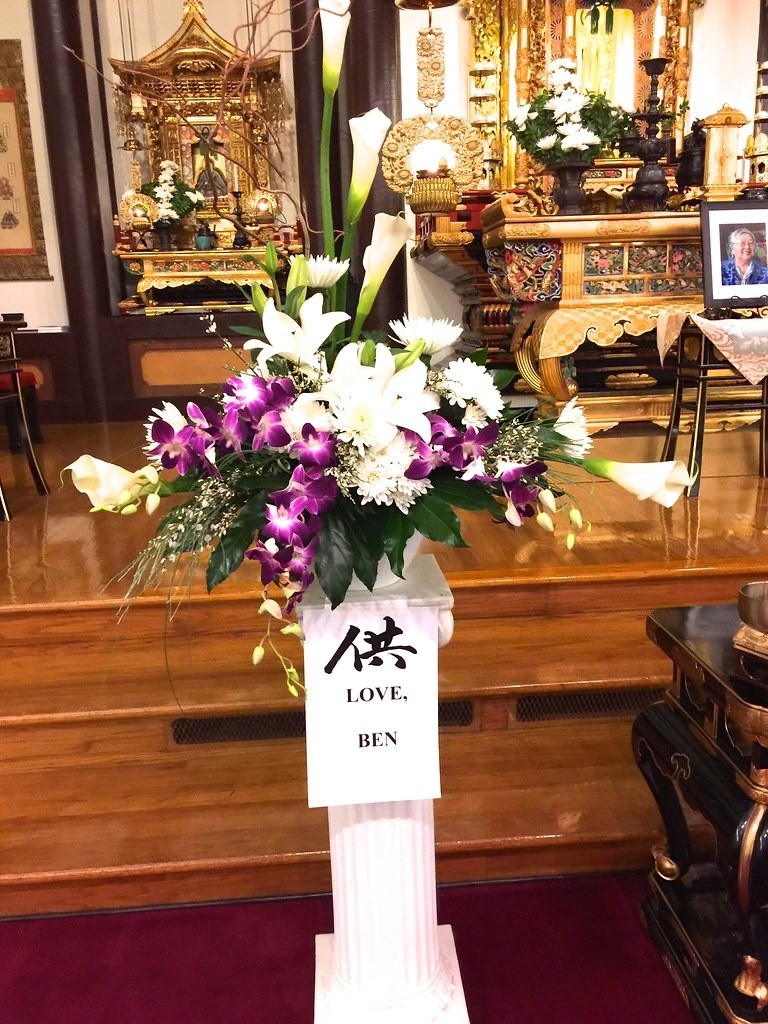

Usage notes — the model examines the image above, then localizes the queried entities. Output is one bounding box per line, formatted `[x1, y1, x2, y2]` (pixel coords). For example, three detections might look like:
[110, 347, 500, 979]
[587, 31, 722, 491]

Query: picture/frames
[702, 200, 768, 307]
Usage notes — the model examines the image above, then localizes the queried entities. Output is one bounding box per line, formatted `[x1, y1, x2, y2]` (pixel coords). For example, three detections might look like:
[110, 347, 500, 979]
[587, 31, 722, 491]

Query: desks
[657, 304, 765, 496]
[631, 604, 767, 1024]
[478, 189, 757, 432]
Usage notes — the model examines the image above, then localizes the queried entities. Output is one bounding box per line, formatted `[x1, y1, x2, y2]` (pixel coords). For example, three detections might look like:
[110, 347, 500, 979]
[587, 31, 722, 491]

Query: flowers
[56, 0, 698, 698]
[492, 60, 631, 164]
[139, 159, 205, 228]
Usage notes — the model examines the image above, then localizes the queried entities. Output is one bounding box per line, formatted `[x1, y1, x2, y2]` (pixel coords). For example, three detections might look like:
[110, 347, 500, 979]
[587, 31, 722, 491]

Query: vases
[149, 219, 177, 251]
[547, 159, 590, 215]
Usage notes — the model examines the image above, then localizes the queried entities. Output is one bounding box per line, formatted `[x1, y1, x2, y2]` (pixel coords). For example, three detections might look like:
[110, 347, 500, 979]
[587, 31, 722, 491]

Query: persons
[721, 227, 768, 286]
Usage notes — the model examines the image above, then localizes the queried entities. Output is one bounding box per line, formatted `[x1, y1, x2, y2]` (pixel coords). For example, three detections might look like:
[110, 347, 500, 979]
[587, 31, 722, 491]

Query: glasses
[732, 241, 755, 247]
